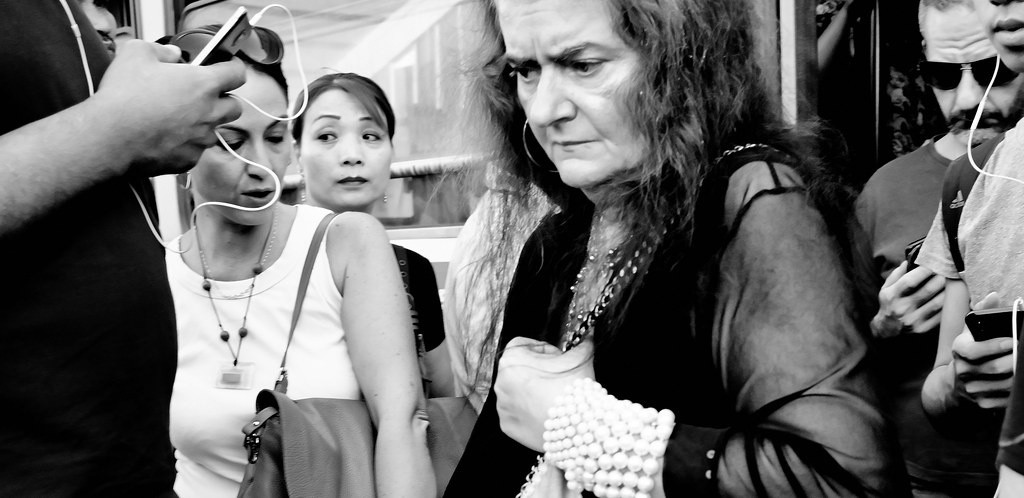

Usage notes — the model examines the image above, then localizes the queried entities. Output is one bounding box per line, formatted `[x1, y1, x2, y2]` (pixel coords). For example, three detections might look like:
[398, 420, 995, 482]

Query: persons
[441, 0, 906, 498]
[920, 0, 1024, 498]
[135, 23, 438, 498]
[73, 0, 121, 62]
[814, 0, 865, 152]
[278, 70, 456, 417]
[0, 0, 249, 498]
[830, 0, 1024, 498]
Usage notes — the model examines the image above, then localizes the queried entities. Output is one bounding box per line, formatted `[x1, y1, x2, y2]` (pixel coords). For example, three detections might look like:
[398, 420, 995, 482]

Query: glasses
[913, 54, 1017, 89]
[95, 27, 133, 46]
[170, 25, 284, 66]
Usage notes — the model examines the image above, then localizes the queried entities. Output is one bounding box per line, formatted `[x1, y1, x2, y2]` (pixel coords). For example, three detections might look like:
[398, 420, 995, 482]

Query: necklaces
[192, 196, 283, 384]
[561, 165, 696, 353]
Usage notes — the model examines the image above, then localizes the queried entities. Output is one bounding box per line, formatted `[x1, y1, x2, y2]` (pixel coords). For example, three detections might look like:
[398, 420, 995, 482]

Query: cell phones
[964, 303, 1024, 343]
[190, 7, 253, 66]
[903, 237, 936, 295]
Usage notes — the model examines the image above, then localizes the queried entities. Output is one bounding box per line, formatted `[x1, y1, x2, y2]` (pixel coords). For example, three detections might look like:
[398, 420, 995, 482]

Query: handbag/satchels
[236, 216, 479, 498]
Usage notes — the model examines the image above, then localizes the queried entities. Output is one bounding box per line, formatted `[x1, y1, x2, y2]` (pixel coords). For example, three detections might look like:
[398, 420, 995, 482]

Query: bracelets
[543, 378, 674, 498]
